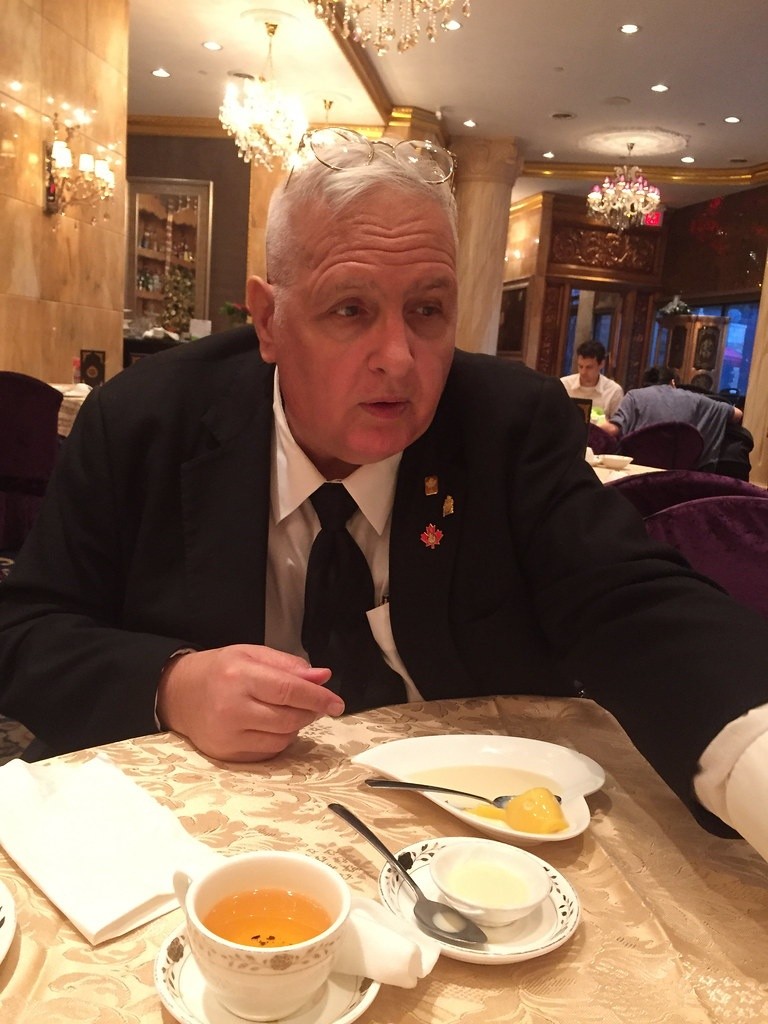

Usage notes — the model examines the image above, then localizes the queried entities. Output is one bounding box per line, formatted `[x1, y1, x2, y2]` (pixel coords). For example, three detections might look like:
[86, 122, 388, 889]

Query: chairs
[586, 422, 618, 455]
[643, 496, 768, 617]
[602, 471, 768, 517]
[0, 370, 65, 562]
[613, 420, 705, 471]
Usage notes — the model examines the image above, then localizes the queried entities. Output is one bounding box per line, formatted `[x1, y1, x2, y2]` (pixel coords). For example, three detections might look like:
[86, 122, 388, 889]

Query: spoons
[328, 802, 488, 943]
[363, 780, 562, 808]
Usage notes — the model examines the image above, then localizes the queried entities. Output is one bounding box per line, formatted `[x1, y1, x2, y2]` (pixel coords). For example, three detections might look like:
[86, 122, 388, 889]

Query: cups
[173, 850, 350, 1021]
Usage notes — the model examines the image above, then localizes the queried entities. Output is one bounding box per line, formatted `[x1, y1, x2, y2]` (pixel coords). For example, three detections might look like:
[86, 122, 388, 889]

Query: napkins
[330, 895, 443, 990]
[0, 751, 228, 947]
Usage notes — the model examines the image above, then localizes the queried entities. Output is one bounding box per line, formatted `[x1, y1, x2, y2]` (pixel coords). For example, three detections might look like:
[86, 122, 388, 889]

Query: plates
[350, 736, 604, 847]
[154, 922, 382, 1024]
[379, 837, 580, 964]
[0, 879, 18, 969]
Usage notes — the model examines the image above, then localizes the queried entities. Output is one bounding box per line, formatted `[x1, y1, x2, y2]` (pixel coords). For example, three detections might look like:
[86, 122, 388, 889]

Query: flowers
[217, 301, 254, 326]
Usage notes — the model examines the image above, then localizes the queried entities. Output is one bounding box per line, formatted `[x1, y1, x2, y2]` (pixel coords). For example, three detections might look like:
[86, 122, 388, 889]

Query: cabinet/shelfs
[123, 176, 214, 334]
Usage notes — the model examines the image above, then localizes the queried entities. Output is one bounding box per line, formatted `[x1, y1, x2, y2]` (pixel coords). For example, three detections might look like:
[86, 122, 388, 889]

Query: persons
[0, 125, 768, 866]
[560, 339, 745, 474]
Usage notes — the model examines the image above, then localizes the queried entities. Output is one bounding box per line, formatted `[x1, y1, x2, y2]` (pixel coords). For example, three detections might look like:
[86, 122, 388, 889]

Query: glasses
[282, 128, 459, 200]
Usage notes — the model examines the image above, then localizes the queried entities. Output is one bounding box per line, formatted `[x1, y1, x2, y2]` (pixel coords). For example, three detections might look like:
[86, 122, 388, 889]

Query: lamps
[217, 7, 310, 175]
[585, 142, 662, 230]
[305, 0, 472, 57]
[44, 140, 116, 234]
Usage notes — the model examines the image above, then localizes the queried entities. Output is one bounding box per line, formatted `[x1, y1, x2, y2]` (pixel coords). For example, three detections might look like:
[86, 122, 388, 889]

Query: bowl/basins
[599, 454, 633, 470]
[431, 850, 551, 929]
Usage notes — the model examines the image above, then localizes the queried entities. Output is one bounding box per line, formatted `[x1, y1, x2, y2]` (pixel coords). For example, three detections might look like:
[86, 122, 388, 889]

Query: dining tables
[584, 447, 668, 485]
[0, 696, 768, 1024]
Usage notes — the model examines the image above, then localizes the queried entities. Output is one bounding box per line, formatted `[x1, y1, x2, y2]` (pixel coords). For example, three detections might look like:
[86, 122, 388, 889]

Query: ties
[300, 484, 409, 714]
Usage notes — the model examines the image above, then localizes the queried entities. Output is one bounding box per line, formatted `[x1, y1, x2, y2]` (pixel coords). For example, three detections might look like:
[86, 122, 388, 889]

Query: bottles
[124, 230, 199, 343]
